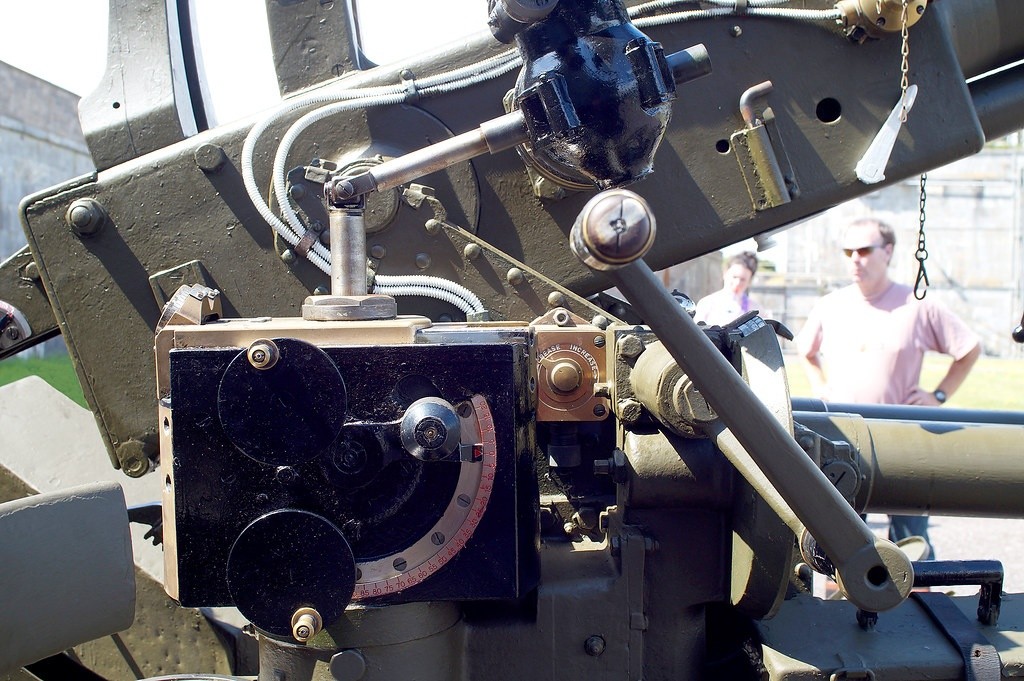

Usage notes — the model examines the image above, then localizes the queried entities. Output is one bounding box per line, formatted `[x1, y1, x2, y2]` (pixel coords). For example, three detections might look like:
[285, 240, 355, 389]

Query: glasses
[841, 245, 884, 258]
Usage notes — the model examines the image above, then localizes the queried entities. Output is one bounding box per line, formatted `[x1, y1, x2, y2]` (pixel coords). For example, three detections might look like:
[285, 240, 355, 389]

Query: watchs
[932, 389, 947, 403]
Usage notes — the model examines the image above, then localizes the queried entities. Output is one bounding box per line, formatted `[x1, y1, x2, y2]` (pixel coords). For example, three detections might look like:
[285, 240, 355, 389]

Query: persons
[795, 217, 983, 564]
[693, 252, 767, 328]
